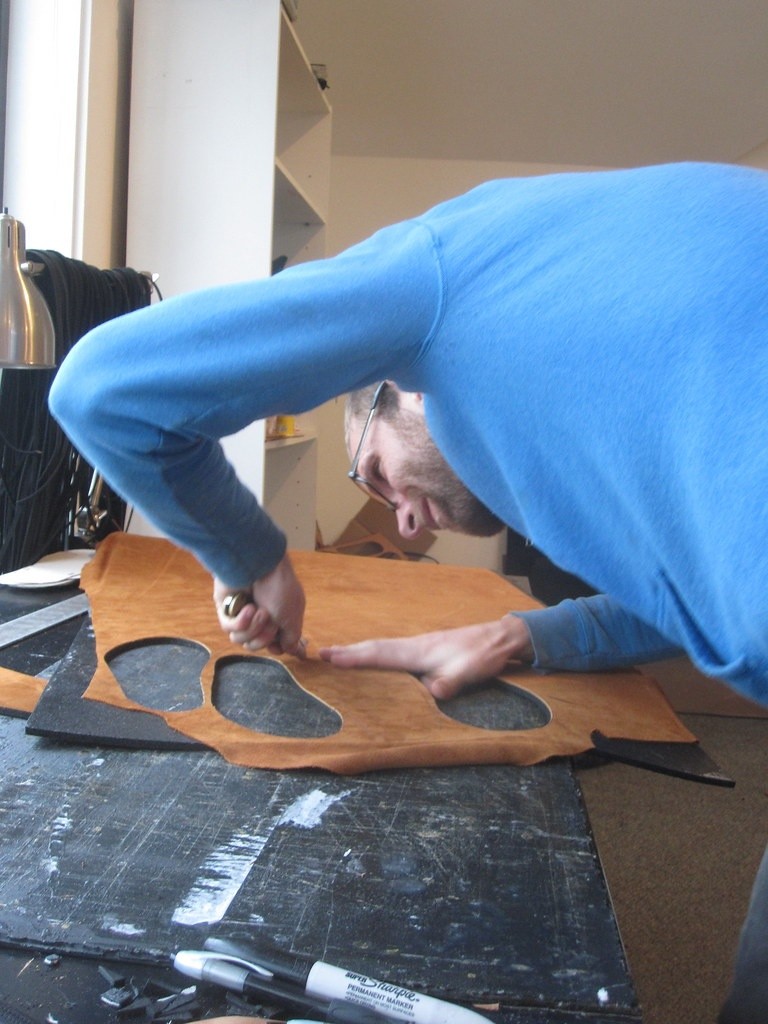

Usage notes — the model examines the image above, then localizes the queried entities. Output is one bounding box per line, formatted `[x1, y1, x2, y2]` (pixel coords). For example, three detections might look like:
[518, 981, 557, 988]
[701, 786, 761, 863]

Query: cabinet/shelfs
[126, 0, 336, 552]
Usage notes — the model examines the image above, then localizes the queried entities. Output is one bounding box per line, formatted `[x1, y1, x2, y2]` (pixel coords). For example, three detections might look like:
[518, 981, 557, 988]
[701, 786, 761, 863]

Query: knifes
[222, 587, 308, 663]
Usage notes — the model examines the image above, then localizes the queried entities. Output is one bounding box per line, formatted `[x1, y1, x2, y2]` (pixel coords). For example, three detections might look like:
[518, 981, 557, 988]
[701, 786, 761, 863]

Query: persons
[47, 162, 768, 1024]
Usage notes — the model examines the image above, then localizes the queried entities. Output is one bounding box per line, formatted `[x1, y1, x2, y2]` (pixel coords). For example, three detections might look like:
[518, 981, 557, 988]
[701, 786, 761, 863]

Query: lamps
[0, 206, 159, 538]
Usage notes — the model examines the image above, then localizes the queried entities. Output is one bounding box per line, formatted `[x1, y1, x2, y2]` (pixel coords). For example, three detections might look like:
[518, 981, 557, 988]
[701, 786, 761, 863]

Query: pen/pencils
[169, 934, 494, 1024]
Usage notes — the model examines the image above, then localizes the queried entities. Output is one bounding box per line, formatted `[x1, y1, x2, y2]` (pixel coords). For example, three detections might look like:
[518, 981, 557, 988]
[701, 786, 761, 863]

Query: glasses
[345, 380, 395, 512]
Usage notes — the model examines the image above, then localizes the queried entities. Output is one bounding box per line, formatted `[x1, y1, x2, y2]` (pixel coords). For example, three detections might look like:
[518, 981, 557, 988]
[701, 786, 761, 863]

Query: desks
[0, 575, 643, 1024]
